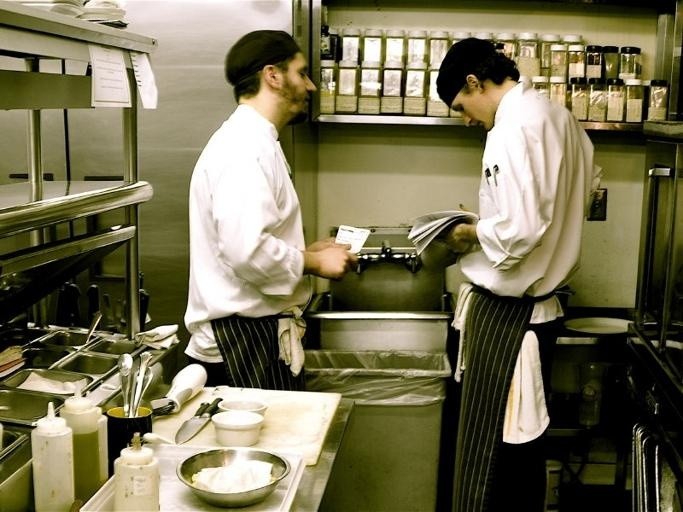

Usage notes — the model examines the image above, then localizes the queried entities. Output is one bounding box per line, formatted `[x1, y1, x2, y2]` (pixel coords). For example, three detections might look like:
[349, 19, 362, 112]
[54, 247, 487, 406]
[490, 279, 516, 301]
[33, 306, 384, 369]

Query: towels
[502, 331, 550, 444]
[134, 324, 180, 351]
[278, 305, 307, 376]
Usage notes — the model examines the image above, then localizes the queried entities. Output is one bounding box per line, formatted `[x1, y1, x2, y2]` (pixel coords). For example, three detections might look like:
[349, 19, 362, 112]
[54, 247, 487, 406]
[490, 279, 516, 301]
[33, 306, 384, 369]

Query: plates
[631, 422, 683, 511]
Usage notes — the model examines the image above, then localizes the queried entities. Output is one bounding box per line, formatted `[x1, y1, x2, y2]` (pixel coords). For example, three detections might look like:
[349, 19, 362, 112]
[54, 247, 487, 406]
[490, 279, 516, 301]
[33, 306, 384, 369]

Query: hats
[436, 38, 505, 108]
[225, 30, 303, 86]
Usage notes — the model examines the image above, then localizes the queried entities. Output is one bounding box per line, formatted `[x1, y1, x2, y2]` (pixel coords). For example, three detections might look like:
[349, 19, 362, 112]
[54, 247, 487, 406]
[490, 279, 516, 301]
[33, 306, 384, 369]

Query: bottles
[321, 25, 331, 58]
[429, 66, 444, 102]
[383, 63, 403, 96]
[60, 388, 101, 502]
[343, 28, 361, 63]
[532, 76, 549, 97]
[320, 60, 337, 95]
[619, 47, 643, 79]
[386, 30, 407, 63]
[99, 408, 108, 487]
[624, 79, 642, 123]
[551, 45, 567, 77]
[540, 33, 561, 68]
[32, 402, 74, 512]
[113, 446, 158, 511]
[471, 33, 493, 42]
[607, 79, 625, 122]
[570, 77, 588, 121]
[329, 29, 339, 61]
[361, 62, 381, 96]
[649, 80, 668, 121]
[338, 62, 358, 96]
[518, 33, 538, 57]
[493, 33, 518, 59]
[568, 45, 586, 86]
[405, 63, 427, 99]
[364, 29, 383, 62]
[428, 30, 451, 65]
[451, 31, 470, 45]
[563, 35, 583, 46]
[642, 80, 650, 121]
[550, 77, 568, 105]
[407, 30, 428, 64]
[603, 46, 619, 80]
[588, 78, 608, 121]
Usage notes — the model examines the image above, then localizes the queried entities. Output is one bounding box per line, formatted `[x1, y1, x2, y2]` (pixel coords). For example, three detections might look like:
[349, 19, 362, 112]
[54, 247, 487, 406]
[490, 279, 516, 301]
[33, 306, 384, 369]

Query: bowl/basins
[50, 351, 119, 375]
[219, 399, 268, 432]
[35, 329, 100, 356]
[19, 347, 76, 377]
[1, 387, 64, 430]
[1, 367, 97, 401]
[0, 427, 28, 462]
[176, 446, 291, 508]
[213, 412, 263, 446]
[81, 337, 148, 358]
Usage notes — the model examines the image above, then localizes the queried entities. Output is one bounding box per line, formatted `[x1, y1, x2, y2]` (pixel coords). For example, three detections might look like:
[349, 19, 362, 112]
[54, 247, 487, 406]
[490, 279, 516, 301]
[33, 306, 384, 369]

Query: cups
[107, 406, 152, 477]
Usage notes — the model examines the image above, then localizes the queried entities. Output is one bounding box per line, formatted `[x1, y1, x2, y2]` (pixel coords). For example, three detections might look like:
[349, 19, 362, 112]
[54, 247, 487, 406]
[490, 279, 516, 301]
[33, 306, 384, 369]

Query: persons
[184, 31, 358, 391]
[432, 38, 595, 512]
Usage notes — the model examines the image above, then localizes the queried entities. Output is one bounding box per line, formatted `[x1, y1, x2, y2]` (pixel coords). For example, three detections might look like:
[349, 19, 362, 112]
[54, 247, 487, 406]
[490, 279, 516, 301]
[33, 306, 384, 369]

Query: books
[21, 0, 130, 29]
[408, 210, 479, 256]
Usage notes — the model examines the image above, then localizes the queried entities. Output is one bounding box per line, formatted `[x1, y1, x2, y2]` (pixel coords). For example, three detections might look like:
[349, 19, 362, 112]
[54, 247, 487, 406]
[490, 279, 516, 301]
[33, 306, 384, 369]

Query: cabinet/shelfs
[315, 0, 683, 340]
[1, 0, 158, 432]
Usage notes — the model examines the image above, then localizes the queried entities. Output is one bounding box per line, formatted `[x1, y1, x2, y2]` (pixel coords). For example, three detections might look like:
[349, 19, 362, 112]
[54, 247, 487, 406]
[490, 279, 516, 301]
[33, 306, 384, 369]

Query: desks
[75, 382, 355, 512]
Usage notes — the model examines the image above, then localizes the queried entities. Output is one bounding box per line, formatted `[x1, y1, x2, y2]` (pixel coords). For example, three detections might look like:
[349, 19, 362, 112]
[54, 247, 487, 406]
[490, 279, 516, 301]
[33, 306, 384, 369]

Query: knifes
[174, 397, 222, 444]
